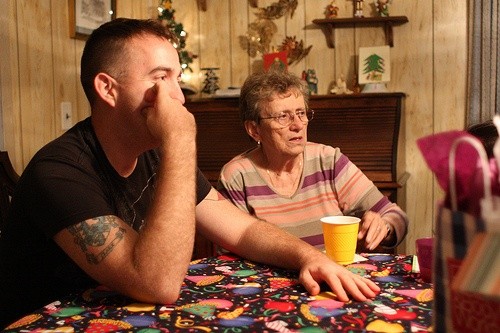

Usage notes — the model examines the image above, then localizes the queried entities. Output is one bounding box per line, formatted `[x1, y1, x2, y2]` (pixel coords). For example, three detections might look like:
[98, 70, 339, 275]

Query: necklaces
[262, 150, 301, 196]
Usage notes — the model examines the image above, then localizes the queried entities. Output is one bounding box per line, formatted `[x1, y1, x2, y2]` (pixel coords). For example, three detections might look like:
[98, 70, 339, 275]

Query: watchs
[384, 219, 391, 242]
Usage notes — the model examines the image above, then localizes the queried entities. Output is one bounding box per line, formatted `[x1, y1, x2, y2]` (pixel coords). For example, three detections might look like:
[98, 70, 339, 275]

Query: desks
[0, 251, 436, 333]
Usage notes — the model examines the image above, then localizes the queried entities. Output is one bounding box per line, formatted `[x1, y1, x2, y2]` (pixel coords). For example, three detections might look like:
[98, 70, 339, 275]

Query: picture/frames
[69, 0, 118, 42]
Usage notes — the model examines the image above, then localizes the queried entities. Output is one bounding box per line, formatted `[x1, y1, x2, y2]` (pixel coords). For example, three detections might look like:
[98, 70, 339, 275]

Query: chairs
[0, 151, 23, 242]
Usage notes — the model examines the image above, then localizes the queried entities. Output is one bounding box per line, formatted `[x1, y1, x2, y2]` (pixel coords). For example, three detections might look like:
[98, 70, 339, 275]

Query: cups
[320, 216, 361, 265]
[416, 238, 434, 283]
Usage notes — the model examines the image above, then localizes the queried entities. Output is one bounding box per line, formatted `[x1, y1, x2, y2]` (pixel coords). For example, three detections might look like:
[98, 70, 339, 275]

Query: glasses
[255, 107, 314, 125]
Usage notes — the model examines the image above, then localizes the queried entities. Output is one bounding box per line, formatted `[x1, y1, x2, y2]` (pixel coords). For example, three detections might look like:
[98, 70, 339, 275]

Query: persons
[220, 72, 407, 253]
[0, 19, 380, 333]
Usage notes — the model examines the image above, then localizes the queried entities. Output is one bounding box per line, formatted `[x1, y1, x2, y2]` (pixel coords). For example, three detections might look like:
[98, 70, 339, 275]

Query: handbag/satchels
[431, 136, 500, 333]
